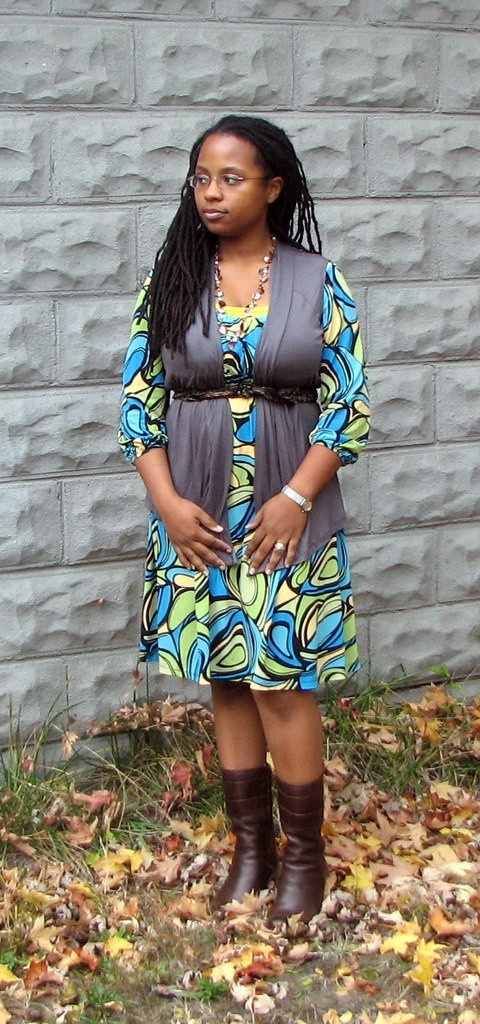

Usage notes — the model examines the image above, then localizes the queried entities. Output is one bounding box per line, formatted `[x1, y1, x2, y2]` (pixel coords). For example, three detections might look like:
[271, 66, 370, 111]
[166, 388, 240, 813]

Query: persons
[117, 117, 371, 930]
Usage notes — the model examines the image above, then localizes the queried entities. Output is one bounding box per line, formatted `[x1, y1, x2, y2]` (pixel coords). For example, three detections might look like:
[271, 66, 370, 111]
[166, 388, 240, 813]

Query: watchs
[281, 485, 312, 513]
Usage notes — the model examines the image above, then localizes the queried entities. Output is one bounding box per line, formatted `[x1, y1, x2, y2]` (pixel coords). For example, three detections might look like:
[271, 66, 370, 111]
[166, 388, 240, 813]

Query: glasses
[188, 174, 266, 190]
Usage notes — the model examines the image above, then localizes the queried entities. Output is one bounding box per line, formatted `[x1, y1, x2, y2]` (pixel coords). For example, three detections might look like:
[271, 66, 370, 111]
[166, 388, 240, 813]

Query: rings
[273, 542, 285, 553]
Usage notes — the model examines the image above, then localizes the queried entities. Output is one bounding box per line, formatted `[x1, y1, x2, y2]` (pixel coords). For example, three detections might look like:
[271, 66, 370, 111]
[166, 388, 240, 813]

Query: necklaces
[210, 235, 276, 342]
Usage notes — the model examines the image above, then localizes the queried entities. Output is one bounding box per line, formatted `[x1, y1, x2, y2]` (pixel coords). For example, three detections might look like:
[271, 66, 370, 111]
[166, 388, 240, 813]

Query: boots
[268, 773, 327, 929]
[210, 763, 278, 912]
